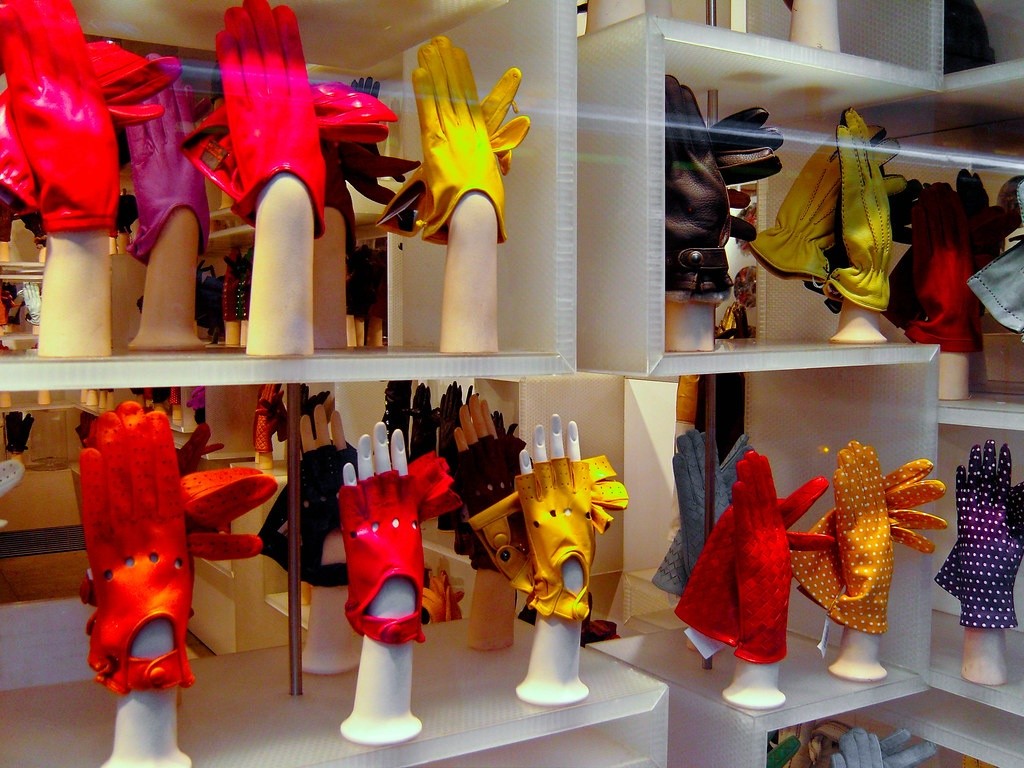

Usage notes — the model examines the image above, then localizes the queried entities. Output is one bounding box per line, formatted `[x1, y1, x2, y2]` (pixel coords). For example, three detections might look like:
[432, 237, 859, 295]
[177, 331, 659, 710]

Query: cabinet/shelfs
[3, 374, 625, 658]
[941, 1, 1023, 768]
[1, 1, 671, 767]
[579, 1, 939, 767]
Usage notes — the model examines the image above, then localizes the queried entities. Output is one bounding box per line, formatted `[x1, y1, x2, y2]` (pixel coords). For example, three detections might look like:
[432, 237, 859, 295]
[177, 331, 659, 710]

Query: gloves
[749, 106, 908, 312]
[91, 386, 204, 422]
[766, 718, 936, 767]
[803, 169, 1023, 353]
[374, 37, 532, 246]
[253, 383, 330, 453]
[790, 440, 946, 634]
[320, 74, 417, 255]
[665, 73, 784, 303]
[416, 568, 467, 625]
[935, 439, 1024, 629]
[650, 428, 755, 595]
[182, 1, 398, 239]
[6, 411, 34, 451]
[674, 450, 835, 664]
[382, 381, 518, 555]
[194, 245, 387, 329]
[0, 278, 43, 326]
[81, 400, 279, 694]
[123, 53, 213, 269]
[0, 0, 181, 243]
